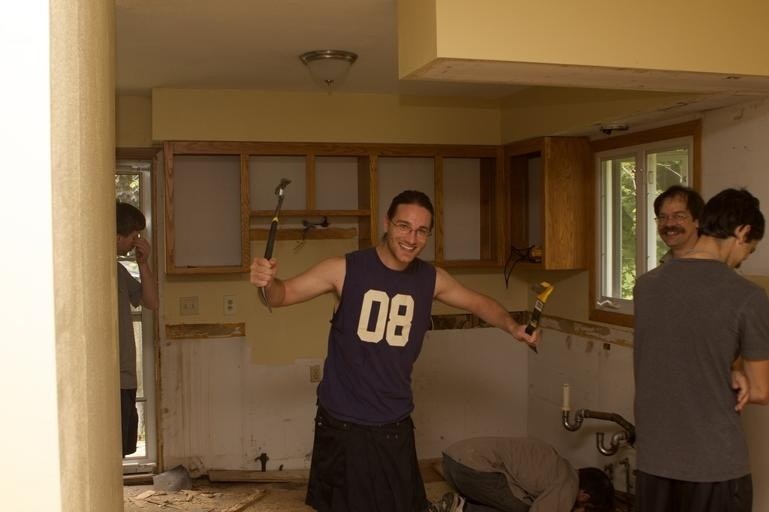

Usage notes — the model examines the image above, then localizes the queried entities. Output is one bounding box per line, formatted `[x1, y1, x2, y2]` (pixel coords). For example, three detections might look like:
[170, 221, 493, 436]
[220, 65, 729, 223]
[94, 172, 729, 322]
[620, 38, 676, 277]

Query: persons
[652, 184, 706, 265]
[248, 187, 541, 511]
[116, 201, 157, 458]
[631, 186, 767, 512]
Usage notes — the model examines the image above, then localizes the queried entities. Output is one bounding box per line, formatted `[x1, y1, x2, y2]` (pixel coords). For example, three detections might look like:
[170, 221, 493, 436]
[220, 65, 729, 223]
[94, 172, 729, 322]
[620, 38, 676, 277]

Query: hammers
[259, 178, 292, 313]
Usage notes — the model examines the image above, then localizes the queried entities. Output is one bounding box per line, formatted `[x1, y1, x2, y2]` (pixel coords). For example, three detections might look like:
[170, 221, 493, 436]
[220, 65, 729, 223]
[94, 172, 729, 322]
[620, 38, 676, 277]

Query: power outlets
[223, 295, 237, 315]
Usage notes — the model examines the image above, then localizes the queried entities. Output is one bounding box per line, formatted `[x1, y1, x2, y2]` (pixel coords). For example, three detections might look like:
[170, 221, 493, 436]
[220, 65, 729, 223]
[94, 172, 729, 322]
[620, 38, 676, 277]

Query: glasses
[652, 214, 692, 225]
[389, 218, 432, 238]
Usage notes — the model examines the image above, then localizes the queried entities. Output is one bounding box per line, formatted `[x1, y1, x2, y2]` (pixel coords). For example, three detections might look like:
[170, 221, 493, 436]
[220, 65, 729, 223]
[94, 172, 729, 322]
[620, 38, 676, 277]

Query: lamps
[301, 51, 358, 91]
